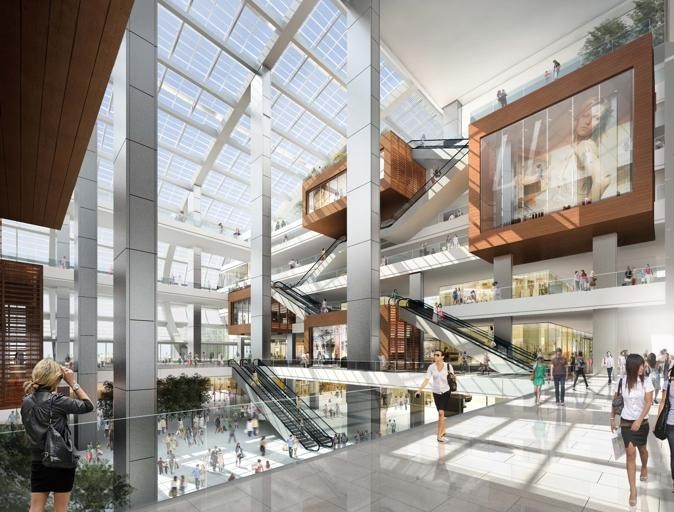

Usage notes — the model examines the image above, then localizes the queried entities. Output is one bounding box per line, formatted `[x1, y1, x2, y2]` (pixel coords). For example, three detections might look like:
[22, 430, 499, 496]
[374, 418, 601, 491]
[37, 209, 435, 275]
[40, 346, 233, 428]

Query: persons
[610, 349, 674, 505]
[414, 350, 455, 442]
[19, 358, 95, 512]
[276, 219, 328, 313]
[531, 348, 630, 405]
[156, 348, 408, 498]
[175, 210, 240, 240]
[61, 256, 66, 269]
[64, 357, 72, 369]
[419, 132, 460, 257]
[390, 289, 495, 374]
[497, 61, 560, 108]
[516, 96, 614, 218]
[452, 264, 654, 304]
[86, 419, 110, 450]
[8, 411, 16, 430]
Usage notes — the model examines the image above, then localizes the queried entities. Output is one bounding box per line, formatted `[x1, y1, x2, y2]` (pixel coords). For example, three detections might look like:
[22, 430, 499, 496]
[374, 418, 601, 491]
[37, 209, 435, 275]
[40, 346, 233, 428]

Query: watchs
[72, 384, 80, 392]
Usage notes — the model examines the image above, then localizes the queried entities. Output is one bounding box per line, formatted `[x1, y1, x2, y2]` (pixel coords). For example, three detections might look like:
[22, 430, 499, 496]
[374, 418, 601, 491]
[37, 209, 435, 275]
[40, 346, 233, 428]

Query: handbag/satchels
[612, 378, 624, 414]
[42, 394, 79, 469]
[612, 429, 625, 458]
[654, 384, 670, 439]
[447, 363, 456, 391]
